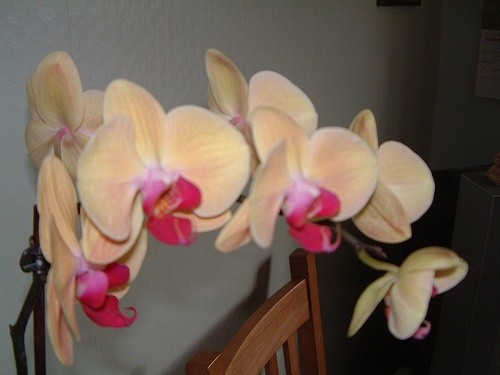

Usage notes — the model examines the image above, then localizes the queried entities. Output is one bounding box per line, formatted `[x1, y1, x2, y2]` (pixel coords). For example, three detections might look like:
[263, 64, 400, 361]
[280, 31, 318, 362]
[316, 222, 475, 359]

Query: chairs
[182, 246, 331, 375]
